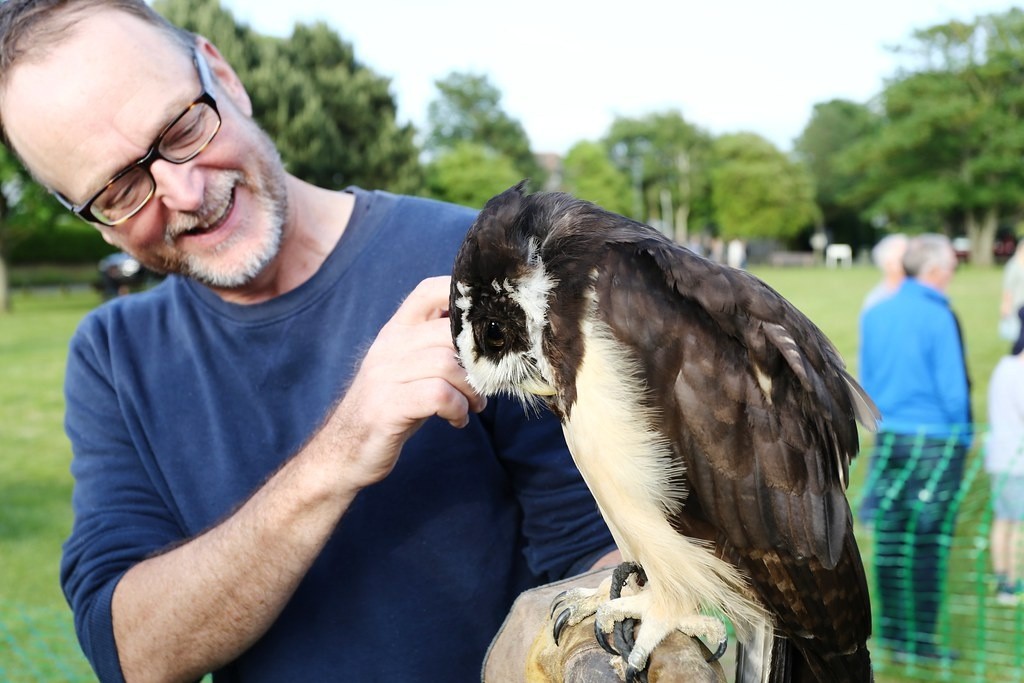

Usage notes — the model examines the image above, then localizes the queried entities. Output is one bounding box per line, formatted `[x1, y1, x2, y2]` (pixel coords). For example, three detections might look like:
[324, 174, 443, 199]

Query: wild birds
[446, 177, 885, 683]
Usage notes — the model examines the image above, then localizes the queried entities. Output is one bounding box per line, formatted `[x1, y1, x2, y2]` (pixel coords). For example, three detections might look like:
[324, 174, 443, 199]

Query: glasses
[50, 47, 222, 228]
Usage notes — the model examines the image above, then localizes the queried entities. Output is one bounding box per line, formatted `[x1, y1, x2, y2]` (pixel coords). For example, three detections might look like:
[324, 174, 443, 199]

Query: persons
[854, 234, 1024, 672]
[0, 0, 731, 683]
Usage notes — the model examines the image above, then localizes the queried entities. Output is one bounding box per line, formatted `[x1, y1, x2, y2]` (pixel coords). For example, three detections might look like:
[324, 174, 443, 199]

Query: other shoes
[914, 646, 957, 665]
[884, 648, 911, 664]
[990, 574, 1024, 606]
